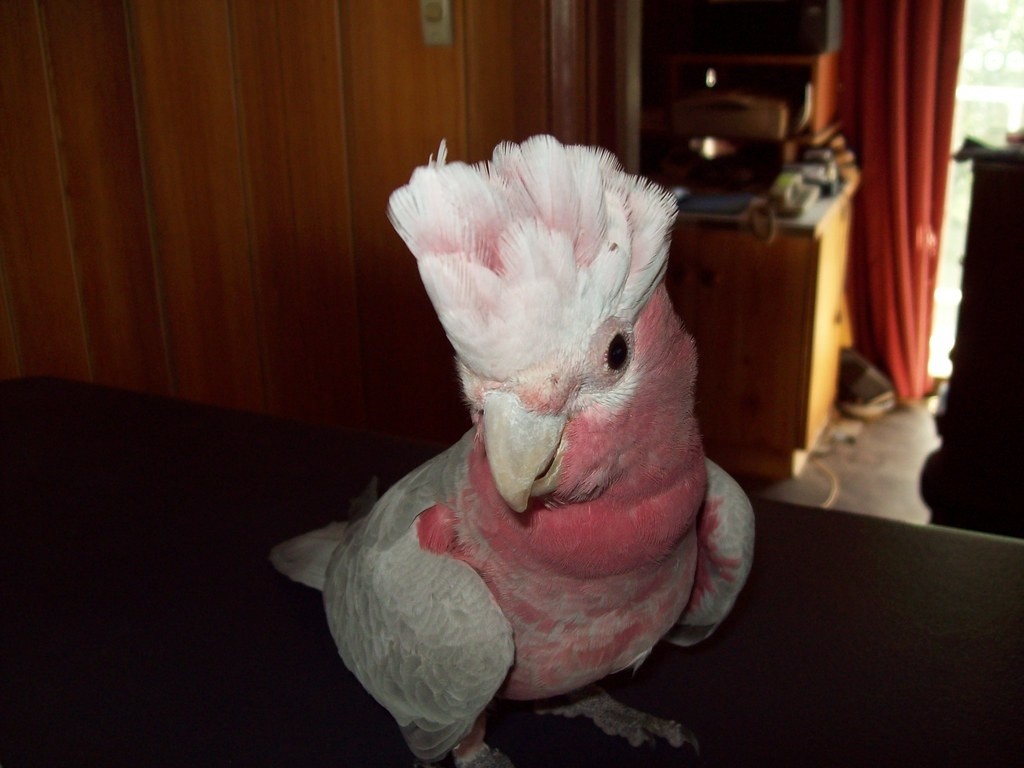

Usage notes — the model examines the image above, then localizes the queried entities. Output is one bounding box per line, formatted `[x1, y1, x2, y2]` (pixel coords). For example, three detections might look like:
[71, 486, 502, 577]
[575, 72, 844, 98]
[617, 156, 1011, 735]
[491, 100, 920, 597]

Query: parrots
[262, 133, 765, 768]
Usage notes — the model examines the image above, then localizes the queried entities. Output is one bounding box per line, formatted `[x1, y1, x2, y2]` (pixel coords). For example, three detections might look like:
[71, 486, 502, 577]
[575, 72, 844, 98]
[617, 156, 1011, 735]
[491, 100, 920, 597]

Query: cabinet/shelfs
[666, 201, 852, 482]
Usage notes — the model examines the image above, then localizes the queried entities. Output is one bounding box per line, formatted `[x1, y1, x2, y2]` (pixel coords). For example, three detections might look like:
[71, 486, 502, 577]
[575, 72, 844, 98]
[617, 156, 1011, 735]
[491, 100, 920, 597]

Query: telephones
[766, 170, 821, 216]
